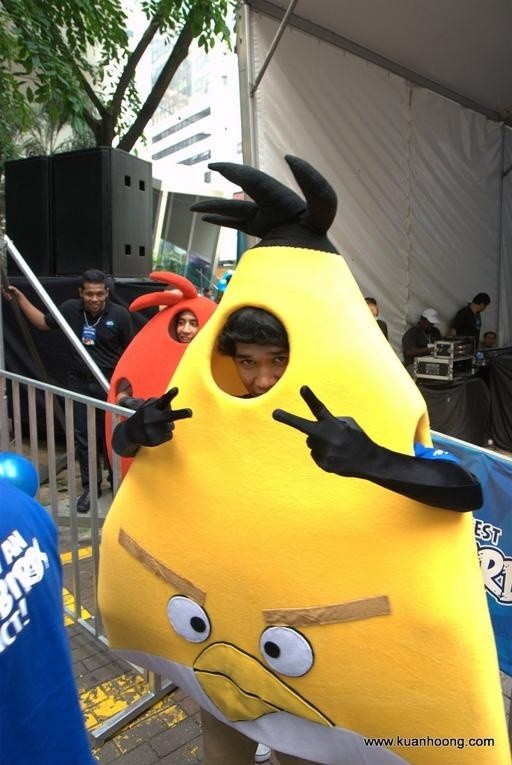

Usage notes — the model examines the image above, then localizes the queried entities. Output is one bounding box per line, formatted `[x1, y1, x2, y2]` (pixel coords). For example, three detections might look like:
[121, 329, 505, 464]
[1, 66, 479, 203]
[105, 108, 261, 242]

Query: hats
[422, 308, 440, 324]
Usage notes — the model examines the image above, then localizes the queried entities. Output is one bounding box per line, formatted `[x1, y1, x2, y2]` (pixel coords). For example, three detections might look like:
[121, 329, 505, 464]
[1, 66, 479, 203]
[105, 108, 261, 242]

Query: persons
[216, 274, 233, 304]
[402, 308, 443, 386]
[483, 332, 498, 347]
[1, 476, 99, 758]
[365, 296, 389, 341]
[202, 286, 213, 298]
[0, 268, 138, 514]
[448, 292, 491, 348]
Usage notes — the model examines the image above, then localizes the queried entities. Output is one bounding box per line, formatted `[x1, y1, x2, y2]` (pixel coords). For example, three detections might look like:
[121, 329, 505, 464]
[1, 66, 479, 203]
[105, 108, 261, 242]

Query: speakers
[4, 155, 53, 275]
[50, 145, 152, 278]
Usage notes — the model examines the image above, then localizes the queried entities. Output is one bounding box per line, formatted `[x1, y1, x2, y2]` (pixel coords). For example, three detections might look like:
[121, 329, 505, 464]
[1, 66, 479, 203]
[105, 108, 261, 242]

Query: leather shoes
[77, 483, 102, 512]
